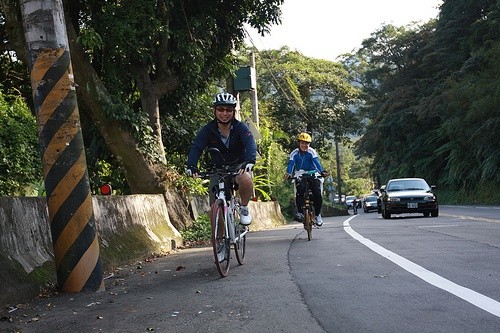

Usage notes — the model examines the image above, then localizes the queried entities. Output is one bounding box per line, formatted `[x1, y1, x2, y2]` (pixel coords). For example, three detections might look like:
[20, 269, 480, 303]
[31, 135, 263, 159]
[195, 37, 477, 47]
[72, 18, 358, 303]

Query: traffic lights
[100, 184, 112, 196]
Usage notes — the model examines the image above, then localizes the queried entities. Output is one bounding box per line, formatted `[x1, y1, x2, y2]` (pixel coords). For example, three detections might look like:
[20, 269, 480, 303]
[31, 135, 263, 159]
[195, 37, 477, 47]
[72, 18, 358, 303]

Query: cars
[333, 194, 360, 208]
[376, 179, 439, 219]
[363, 196, 380, 213]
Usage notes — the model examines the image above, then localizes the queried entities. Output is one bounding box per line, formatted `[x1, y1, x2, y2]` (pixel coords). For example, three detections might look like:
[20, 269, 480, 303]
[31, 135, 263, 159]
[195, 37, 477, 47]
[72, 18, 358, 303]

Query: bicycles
[284, 174, 330, 241]
[188, 167, 254, 278]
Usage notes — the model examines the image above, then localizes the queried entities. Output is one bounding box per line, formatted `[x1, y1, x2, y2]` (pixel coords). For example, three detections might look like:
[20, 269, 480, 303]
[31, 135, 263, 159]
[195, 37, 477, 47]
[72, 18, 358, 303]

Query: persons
[352, 198, 358, 212]
[285, 133, 327, 226]
[186, 93, 256, 263]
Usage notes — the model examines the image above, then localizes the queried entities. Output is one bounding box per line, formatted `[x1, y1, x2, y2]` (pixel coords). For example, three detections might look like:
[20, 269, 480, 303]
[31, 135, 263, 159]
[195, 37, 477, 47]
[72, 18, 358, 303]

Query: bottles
[228, 205, 233, 222]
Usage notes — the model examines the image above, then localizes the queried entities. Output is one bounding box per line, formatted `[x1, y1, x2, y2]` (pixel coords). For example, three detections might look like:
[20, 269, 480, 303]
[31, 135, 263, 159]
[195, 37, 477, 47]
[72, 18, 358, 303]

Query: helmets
[296, 132, 312, 142]
[212, 93, 237, 105]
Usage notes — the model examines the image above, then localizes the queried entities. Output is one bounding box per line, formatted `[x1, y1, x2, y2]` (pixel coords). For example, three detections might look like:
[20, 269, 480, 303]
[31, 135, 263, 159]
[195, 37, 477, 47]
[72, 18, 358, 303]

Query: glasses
[215, 106, 235, 112]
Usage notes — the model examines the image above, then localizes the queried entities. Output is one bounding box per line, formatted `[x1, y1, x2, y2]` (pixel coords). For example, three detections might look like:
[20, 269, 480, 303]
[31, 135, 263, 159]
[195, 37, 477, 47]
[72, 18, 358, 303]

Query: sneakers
[296, 212, 302, 219]
[315, 214, 322, 226]
[213, 243, 224, 263]
[239, 205, 251, 225]
